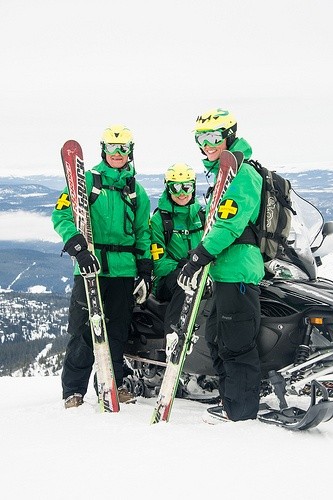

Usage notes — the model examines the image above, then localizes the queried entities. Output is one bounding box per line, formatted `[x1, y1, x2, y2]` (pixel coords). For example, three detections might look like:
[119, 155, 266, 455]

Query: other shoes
[117, 386, 138, 405]
[64, 395, 83, 409]
[202, 405, 233, 426]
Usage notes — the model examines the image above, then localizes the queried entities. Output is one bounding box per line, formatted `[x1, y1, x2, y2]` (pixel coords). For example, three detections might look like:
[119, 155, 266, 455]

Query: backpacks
[205, 159, 297, 263]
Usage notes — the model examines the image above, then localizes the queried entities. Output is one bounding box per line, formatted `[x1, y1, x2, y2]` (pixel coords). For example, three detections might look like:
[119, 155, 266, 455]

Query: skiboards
[61, 140, 121, 414]
[153, 150, 245, 422]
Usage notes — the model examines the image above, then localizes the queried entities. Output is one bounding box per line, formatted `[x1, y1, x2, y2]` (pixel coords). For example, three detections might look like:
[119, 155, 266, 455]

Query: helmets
[193, 108, 237, 147]
[100, 124, 134, 160]
[164, 164, 197, 198]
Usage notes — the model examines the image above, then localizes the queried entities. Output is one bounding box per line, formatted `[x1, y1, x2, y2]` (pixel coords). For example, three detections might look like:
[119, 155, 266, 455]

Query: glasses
[164, 178, 196, 196]
[100, 143, 133, 156]
[194, 122, 238, 148]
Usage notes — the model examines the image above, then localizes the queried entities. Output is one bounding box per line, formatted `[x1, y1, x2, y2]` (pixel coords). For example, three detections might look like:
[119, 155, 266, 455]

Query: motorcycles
[121, 252, 333, 433]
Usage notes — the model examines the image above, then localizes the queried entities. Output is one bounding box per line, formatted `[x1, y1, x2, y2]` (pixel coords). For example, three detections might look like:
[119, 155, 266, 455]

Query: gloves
[177, 242, 215, 291]
[132, 258, 153, 305]
[61, 233, 101, 277]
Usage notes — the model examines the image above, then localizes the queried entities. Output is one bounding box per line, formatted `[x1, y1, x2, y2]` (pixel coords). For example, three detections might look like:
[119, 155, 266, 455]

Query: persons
[175, 109, 268, 424]
[150, 163, 207, 365]
[50, 126, 152, 409]
